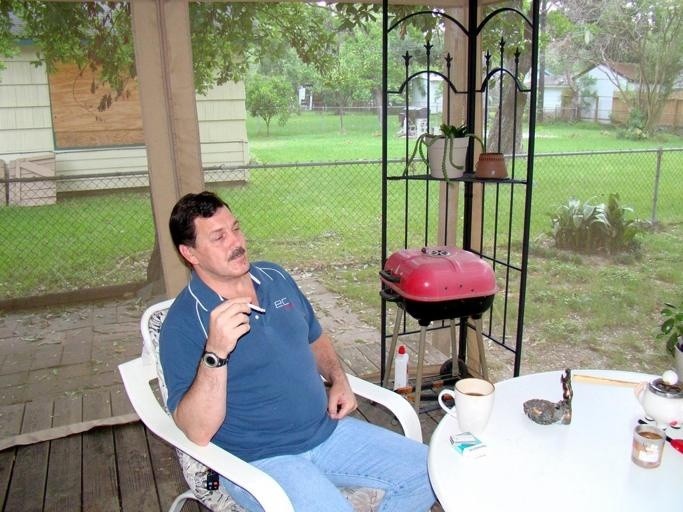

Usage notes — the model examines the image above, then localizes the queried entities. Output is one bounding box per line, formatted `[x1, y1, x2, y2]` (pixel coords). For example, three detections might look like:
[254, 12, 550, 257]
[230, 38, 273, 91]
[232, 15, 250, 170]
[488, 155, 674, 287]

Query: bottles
[392, 343, 408, 390]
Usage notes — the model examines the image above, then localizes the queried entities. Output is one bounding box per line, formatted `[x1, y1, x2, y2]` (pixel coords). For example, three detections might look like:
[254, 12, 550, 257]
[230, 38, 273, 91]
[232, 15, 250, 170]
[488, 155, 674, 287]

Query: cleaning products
[393, 345, 410, 392]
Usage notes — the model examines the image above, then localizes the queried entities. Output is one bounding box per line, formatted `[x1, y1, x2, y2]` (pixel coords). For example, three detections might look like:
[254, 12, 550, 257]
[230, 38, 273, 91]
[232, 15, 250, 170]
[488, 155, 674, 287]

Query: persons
[159, 190, 439, 511]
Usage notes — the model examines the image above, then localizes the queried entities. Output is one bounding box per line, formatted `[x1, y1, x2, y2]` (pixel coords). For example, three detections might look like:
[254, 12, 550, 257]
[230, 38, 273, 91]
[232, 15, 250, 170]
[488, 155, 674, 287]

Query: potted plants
[655, 302, 682, 379]
[402, 123, 485, 188]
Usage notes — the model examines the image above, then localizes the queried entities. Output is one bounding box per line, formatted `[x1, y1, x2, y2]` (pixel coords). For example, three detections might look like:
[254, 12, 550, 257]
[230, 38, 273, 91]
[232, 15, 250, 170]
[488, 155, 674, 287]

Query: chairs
[116, 298, 424, 512]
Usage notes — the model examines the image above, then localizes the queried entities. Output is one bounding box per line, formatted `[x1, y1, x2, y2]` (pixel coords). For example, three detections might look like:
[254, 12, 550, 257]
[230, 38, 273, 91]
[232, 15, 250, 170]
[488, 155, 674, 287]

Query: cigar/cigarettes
[248, 303, 268, 315]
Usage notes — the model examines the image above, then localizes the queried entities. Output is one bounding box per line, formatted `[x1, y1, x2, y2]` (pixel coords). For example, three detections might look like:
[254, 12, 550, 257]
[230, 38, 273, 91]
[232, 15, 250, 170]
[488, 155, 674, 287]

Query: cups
[631, 425, 665, 468]
[634, 370, 683, 425]
[437, 376, 495, 430]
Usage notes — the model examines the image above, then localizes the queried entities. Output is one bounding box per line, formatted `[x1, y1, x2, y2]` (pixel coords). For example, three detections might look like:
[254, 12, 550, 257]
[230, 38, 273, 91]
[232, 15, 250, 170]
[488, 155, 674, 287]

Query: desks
[427, 369, 682, 512]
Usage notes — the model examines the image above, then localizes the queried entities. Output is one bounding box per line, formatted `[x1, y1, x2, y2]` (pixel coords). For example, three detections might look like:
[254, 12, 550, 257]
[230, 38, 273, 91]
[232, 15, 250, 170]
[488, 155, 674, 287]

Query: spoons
[637, 418, 683, 454]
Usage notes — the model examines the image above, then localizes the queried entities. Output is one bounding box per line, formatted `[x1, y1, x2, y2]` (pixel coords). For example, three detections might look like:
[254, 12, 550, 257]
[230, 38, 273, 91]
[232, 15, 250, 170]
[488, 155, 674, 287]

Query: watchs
[202, 348, 229, 369]
